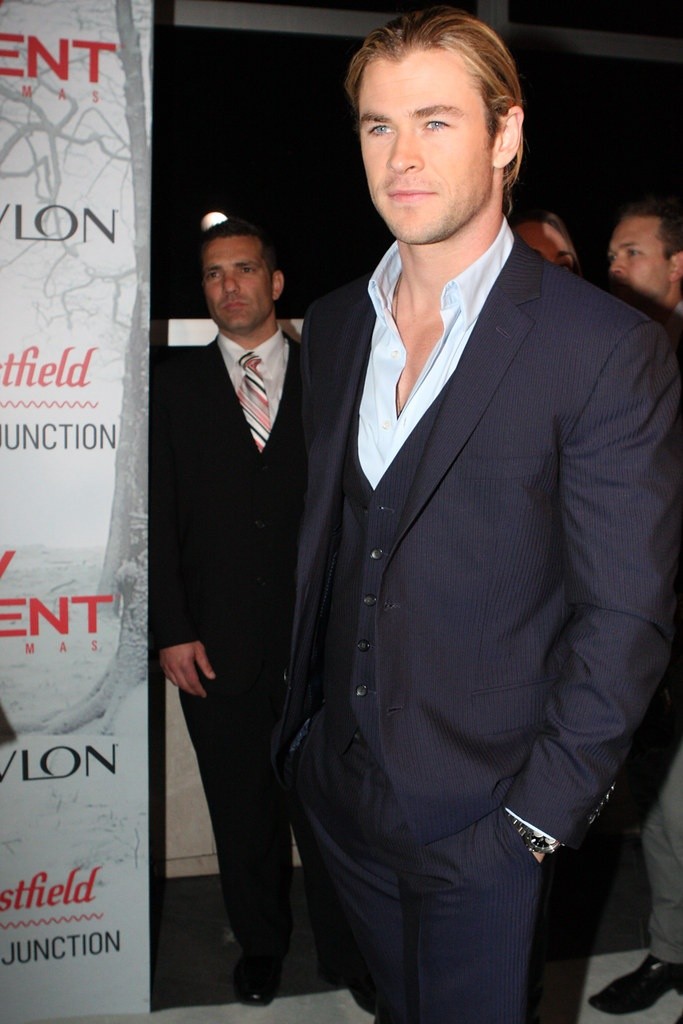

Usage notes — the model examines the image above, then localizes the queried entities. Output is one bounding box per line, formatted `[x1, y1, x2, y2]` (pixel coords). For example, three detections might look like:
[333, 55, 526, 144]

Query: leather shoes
[232, 942, 292, 1007]
[587, 954, 683, 1013]
[318, 954, 381, 1016]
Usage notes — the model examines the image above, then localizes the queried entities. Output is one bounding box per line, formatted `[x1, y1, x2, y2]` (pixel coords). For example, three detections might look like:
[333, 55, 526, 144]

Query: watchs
[505, 812, 560, 854]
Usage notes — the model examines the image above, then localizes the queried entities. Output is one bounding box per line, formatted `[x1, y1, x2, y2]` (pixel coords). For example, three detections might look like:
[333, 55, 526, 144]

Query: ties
[238, 351, 271, 452]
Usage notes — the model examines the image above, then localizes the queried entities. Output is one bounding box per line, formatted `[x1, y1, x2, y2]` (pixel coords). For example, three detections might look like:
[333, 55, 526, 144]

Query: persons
[507, 208, 583, 279]
[587, 197, 683, 1024]
[149, 218, 396, 1017]
[260, 4, 683, 1023]
